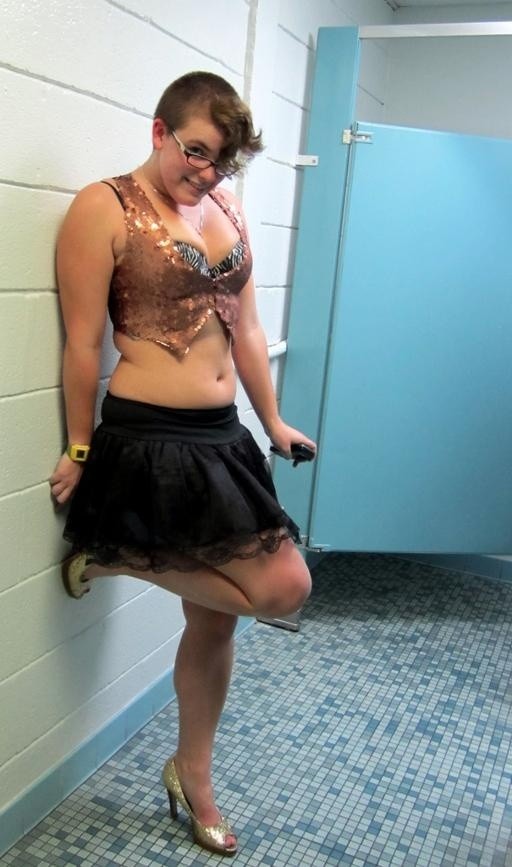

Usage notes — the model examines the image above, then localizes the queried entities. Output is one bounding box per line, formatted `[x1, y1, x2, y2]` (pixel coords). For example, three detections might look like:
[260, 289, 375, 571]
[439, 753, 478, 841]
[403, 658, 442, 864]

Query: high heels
[160, 748, 240, 858]
[64, 549, 96, 599]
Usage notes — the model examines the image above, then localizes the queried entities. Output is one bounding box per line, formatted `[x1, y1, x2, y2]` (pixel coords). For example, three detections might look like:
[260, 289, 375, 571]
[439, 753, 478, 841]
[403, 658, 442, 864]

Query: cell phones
[270, 444, 316, 461]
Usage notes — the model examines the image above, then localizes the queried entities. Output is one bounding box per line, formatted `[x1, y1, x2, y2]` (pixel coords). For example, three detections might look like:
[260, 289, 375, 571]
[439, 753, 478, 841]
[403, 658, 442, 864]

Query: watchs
[64, 441, 90, 464]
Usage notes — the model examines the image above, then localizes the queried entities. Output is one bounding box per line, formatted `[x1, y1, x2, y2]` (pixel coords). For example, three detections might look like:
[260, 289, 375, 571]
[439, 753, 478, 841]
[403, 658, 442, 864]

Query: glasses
[166, 127, 242, 178]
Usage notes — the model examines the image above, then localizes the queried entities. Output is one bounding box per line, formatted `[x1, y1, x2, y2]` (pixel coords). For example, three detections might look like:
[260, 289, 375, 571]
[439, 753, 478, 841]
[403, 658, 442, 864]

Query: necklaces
[139, 164, 204, 237]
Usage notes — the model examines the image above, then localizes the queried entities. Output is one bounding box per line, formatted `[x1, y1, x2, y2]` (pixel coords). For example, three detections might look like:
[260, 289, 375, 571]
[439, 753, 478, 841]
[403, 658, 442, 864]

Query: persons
[48, 70, 320, 857]
